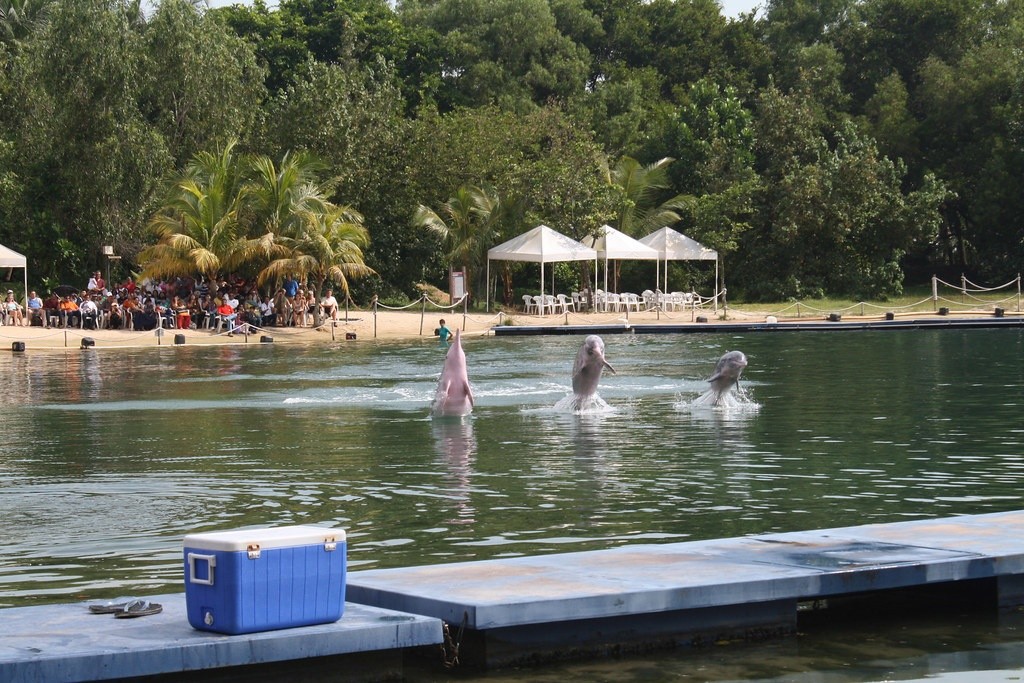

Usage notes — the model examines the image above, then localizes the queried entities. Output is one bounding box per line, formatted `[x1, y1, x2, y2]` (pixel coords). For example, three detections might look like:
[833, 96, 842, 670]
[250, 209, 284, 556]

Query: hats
[300, 290, 303, 293]
[119, 288, 128, 294]
[8, 290, 13, 293]
[111, 303, 117, 307]
[107, 292, 112, 296]
[309, 291, 313, 294]
[194, 291, 200, 295]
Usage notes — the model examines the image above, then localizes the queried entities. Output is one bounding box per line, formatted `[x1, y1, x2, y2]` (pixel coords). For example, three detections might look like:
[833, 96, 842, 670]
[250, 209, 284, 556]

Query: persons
[439, 319, 453, 340]
[0, 269, 338, 337]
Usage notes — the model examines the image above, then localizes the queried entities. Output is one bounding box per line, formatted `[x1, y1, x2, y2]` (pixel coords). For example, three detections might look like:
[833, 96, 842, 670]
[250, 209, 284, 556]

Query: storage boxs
[184, 524, 347, 636]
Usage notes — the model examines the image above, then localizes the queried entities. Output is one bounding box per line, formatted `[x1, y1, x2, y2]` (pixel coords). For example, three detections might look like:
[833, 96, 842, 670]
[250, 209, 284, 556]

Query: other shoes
[229, 332, 233, 337]
[60, 325, 64, 328]
[170, 324, 174, 328]
[211, 327, 215, 330]
[68, 325, 71, 327]
[334, 322, 337, 327]
[47, 325, 51, 328]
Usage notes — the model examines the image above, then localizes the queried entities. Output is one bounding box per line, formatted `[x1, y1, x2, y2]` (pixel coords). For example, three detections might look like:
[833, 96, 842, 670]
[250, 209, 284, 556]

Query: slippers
[89, 599, 145, 613]
[115, 600, 162, 616]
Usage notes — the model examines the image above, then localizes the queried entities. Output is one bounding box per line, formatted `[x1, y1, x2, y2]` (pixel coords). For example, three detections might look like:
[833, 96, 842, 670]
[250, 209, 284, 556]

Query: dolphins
[433, 328, 474, 416]
[707, 351, 748, 406]
[571, 334, 616, 410]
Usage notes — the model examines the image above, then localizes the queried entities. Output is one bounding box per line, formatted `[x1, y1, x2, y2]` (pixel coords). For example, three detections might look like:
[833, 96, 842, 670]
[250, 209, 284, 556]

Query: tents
[0, 244, 29, 326]
[486, 225, 718, 313]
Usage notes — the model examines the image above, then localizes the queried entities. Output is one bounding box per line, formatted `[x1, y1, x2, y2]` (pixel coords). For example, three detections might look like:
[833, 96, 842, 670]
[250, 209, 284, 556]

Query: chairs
[521, 289, 703, 316]
[27, 304, 339, 334]
[1, 309, 14, 326]
